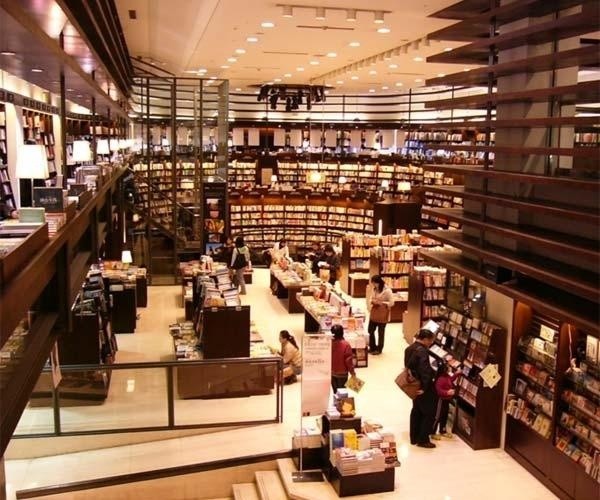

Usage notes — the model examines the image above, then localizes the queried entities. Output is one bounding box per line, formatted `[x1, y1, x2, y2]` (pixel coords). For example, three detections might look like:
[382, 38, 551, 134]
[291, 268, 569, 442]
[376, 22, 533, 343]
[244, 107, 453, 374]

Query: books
[0, 111, 599, 486]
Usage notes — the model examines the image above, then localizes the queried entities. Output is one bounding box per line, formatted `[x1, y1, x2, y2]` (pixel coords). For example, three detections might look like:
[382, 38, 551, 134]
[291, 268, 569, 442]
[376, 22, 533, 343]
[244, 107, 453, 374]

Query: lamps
[256, 83, 334, 113]
[275, 3, 394, 24]
[309, 32, 442, 84]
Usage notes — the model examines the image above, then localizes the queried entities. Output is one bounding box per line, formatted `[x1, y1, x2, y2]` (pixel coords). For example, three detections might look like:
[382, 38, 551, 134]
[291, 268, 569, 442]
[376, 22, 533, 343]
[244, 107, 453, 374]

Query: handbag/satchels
[370, 304, 388, 323]
[395, 366, 421, 400]
[227, 254, 246, 269]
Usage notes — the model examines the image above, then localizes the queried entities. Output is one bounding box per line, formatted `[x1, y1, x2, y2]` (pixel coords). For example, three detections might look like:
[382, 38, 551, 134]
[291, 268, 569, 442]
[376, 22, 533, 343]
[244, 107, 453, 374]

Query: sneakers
[432, 434, 440, 440]
[440, 432, 452, 438]
[418, 441, 435, 447]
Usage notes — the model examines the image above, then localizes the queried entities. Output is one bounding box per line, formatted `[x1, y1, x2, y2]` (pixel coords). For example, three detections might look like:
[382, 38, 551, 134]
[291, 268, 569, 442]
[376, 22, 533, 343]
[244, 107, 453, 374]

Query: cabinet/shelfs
[0, 86, 132, 285]
[270, 269, 374, 368]
[131, 144, 462, 268]
[506, 298, 599, 499]
[286, 408, 398, 496]
[395, 268, 505, 454]
[334, 231, 415, 322]
[34, 269, 287, 400]
